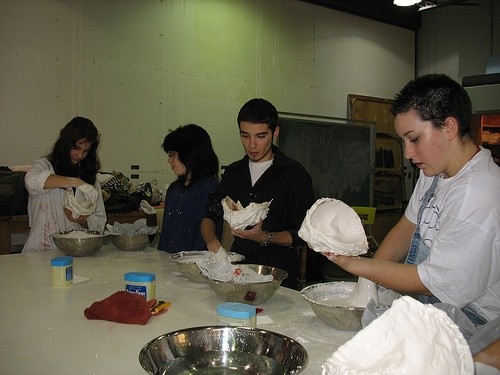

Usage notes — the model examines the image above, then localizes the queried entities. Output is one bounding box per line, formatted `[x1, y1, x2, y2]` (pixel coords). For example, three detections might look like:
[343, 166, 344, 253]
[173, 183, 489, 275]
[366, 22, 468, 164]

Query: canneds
[123, 272, 156, 303]
[50, 256, 73, 288]
[217, 301, 256, 327]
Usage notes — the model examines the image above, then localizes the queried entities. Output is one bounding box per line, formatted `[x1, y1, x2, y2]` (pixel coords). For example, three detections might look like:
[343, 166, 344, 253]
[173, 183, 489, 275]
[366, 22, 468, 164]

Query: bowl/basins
[52, 229, 108, 257]
[109, 229, 156, 251]
[170, 249, 246, 282]
[300, 281, 402, 332]
[202, 262, 288, 305]
[137, 325, 309, 375]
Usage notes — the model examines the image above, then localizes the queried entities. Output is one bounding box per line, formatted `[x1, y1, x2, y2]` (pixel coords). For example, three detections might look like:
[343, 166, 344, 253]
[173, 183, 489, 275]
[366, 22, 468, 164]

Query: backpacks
[96, 171, 153, 213]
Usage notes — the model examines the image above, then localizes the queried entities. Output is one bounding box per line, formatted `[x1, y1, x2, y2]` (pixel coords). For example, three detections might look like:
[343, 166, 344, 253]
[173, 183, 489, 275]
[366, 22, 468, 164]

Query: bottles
[216, 301, 257, 330]
[50, 257, 73, 286]
[122, 271, 157, 303]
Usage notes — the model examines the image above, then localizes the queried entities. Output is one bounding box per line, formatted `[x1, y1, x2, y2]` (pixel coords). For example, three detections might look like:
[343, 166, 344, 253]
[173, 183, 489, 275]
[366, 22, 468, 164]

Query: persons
[200, 98, 314, 290]
[21, 116, 107, 254]
[157, 124, 224, 254]
[321, 74, 500, 329]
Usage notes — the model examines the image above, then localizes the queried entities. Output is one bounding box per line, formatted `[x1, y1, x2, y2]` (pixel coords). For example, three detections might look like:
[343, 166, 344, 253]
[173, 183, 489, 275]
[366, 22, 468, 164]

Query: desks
[0, 243, 357, 375]
[0, 211, 145, 254]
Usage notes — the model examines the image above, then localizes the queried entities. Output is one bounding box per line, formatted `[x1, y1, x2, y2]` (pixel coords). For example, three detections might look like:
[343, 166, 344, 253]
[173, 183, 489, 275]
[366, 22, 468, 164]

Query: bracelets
[259, 232, 272, 247]
